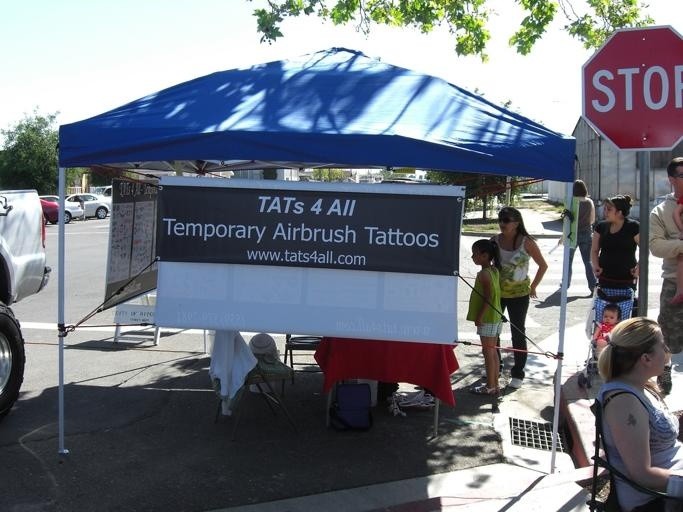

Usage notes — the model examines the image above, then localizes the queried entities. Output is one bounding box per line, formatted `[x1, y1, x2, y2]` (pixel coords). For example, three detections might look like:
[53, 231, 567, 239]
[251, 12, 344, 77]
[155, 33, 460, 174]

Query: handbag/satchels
[329, 383, 375, 432]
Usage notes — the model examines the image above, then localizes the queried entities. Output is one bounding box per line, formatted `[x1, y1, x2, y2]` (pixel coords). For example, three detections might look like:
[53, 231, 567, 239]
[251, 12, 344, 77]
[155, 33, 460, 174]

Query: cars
[40, 185, 114, 227]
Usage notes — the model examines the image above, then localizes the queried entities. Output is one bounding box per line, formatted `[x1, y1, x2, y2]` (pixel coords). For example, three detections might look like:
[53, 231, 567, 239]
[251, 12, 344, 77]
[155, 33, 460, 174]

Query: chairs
[210, 331, 297, 441]
[588, 397, 683, 512]
[281, 334, 323, 397]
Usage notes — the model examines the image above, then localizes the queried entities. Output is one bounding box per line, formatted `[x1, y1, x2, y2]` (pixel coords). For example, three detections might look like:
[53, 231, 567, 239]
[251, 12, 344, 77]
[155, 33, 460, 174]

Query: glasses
[674, 174, 683, 179]
[497, 217, 518, 225]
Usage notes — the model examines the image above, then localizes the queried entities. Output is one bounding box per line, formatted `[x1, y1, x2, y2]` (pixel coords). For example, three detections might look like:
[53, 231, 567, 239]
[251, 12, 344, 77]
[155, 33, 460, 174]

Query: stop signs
[579, 24, 683, 152]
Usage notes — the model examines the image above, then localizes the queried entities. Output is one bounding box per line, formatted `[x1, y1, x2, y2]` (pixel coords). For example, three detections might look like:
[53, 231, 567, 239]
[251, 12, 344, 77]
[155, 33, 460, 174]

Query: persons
[591, 196, 640, 288]
[465, 239, 503, 395]
[592, 304, 622, 359]
[668, 195, 683, 305]
[597, 317, 683, 511]
[649, 156, 683, 395]
[481, 206, 548, 389]
[558, 179, 597, 297]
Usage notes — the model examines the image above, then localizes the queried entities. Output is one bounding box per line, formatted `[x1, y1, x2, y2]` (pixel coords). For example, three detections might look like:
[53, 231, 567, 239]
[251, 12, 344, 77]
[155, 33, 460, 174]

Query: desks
[324, 337, 451, 436]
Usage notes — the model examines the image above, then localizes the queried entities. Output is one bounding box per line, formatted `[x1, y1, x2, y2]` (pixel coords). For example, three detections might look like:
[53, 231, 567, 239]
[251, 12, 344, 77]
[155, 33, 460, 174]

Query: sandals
[469, 385, 500, 395]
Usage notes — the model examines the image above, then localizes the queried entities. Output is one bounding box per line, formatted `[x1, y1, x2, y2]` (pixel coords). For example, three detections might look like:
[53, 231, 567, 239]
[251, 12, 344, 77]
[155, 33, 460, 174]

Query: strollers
[578, 269, 636, 388]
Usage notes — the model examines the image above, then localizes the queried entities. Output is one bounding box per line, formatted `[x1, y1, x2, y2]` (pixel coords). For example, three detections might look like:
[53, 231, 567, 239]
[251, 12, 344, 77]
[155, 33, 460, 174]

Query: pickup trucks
[0, 185, 53, 428]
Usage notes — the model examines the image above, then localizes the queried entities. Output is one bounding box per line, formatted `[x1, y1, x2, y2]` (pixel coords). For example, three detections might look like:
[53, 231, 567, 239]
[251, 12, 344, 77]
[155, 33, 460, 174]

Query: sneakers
[669, 294, 683, 310]
[481, 368, 503, 378]
[508, 376, 523, 389]
[656, 364, 674, 395]
[400, 389, 436, 410]
